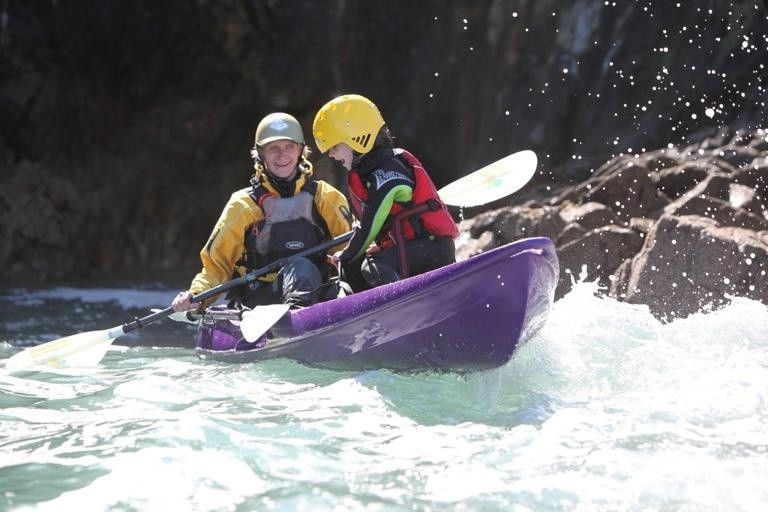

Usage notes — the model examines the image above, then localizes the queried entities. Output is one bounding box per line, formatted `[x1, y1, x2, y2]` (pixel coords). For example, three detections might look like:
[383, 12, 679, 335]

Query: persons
[172, 112, 359, 312]
[313, 94, 460, 294]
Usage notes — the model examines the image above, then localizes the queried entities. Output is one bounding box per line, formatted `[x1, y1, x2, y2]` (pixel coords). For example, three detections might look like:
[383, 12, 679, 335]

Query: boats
[196, 236, 561, 374]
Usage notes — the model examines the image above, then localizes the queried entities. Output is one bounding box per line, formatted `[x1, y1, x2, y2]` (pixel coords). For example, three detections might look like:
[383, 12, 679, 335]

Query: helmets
[254, 111, 305, 147]
[312, 94, 386, 154]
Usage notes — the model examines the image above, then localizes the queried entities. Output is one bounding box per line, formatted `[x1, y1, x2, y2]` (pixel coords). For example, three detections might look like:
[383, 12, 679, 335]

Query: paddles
[151, 304, 291, 343]
[4, 149, 538, 374]
